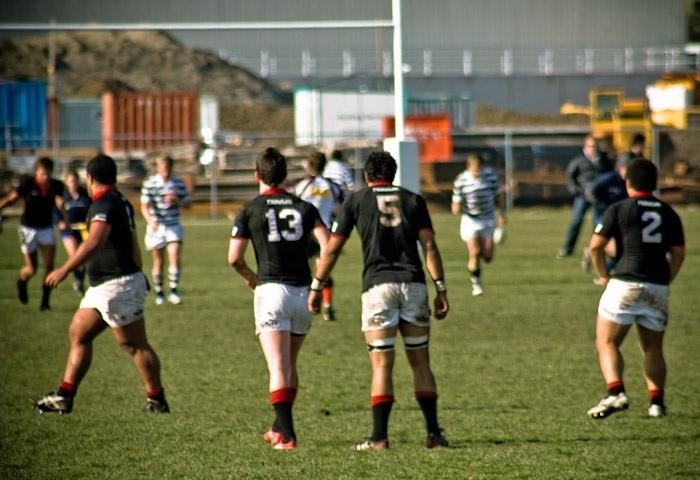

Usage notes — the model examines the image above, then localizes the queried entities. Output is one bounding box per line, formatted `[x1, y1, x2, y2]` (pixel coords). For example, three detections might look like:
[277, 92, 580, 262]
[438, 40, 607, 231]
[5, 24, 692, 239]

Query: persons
[588, 159, 685, 420]
[294, 148, 355, 322]
[31, 153, 171, 416]
[228, 146, 330, 448]
[559, 132, 649, 274]
[1, 157, 91, 312]
[452, 154, 505, 295]
[139, 155, 192, 304]
[308, 152, 450, 449]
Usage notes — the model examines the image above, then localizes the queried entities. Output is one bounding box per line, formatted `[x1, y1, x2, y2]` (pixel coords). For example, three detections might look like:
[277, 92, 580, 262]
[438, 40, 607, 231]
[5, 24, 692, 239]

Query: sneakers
[471, 280, 484, 296]
[154, 293, 182, 306]
[273, 439, 296, 450]
[426, 433, 450, 448]
[17, 268, 89, 314]
[648, 404, 667, 418]
[263, 429, 281, 443]
[138, 400, 170, 414]
[587, 392, 630, 419]
[31, 394, 73, 414]
[356, 436, 390, 452]
[322, 305, 335, 322]
